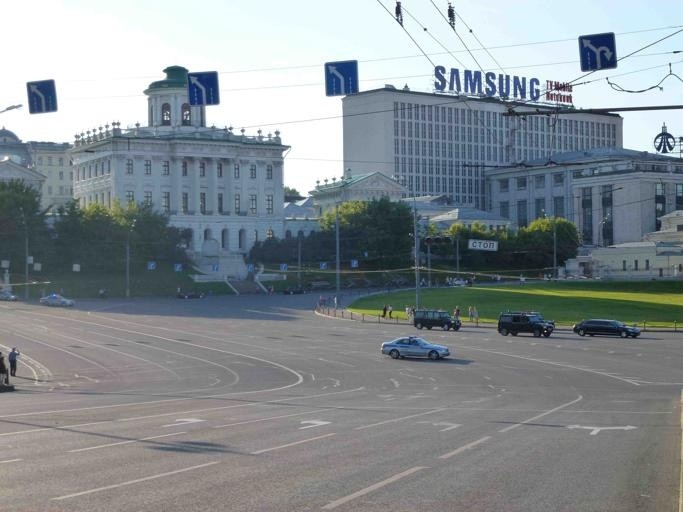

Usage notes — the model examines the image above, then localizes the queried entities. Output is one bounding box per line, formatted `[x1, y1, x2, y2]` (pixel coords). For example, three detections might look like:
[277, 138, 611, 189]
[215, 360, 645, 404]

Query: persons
[380, 302, 480, 326]
[0, 356, 8, 384]
[418, 273, 478, 288]
[7, 348, 18, 378]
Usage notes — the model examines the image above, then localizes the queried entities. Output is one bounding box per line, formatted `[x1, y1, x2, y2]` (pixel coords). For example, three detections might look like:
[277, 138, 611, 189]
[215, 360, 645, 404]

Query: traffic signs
[467, 239, 498, 252]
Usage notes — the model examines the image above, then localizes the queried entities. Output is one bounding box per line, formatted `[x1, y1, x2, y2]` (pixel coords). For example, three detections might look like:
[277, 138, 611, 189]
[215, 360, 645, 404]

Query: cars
[178, 290, 203, 299]
[0, 287, 18, 301]
[552, 273, 603, 280]
[573, 319, 642, 338]
[284, 285, 307, 295]
[382, 335, 451, 360]
[40, 294, 75, 308]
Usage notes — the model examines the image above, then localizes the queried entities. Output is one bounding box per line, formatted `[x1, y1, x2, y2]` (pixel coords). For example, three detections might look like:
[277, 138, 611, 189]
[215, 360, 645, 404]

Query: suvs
[497, 310, 557, 336]
[411, 309, 462, 330]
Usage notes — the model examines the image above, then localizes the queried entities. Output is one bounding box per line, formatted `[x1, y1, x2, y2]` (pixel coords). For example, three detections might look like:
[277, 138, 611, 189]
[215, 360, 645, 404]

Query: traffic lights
[424, 235, 453, 244]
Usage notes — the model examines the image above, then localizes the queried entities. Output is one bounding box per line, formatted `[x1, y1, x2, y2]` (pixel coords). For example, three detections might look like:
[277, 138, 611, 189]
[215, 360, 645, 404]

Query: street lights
[540, 208, 557, 282]
[17, 205, 31, 302]
[328, 202, 342, 302]
[126, 216, 138, 298]
